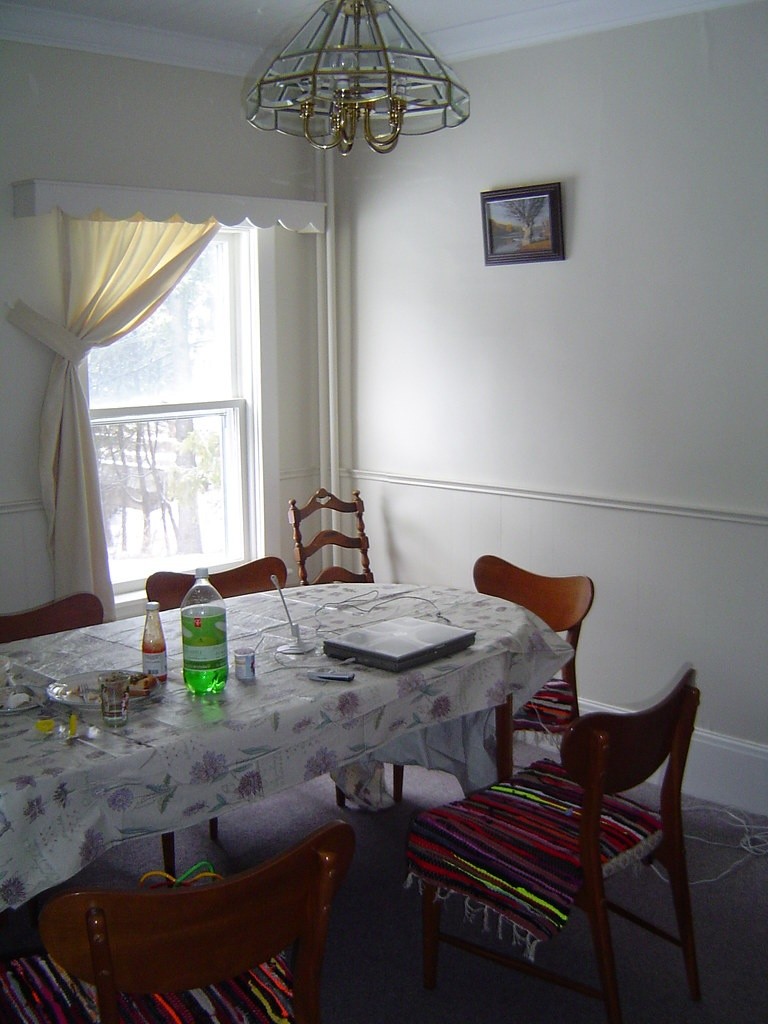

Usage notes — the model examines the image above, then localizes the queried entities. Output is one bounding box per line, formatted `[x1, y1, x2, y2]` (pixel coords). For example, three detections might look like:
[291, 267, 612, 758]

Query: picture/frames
[477, 180, 565, 267]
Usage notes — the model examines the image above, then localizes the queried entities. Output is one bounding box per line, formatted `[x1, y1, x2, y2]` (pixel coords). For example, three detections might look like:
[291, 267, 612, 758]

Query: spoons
[14, 685, 60, 715]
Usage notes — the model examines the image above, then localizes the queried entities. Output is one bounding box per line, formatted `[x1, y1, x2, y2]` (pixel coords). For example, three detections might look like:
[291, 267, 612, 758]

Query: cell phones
[307, 671, 356, 682]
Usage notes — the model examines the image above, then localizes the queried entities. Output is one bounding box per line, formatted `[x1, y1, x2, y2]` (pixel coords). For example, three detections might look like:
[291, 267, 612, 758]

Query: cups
[98, 673, 130, 727]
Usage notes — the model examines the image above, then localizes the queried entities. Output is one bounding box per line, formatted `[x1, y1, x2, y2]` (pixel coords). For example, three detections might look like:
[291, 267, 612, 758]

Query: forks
[77, 682, 90, 704]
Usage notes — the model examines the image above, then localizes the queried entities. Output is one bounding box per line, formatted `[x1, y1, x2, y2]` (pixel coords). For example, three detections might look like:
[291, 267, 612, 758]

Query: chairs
[0, 591, 177, 890]
[0, 817, 358, 1024]
[144, 556, 346, 841]
[472, 554, 594, 736]
[401, 667, 703, 1024]
[287, 487, 374, 584]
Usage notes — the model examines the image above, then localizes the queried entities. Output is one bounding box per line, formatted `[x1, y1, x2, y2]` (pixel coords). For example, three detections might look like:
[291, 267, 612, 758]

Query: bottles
[180, 568, 229, 696]
[142, 602, 168, 684]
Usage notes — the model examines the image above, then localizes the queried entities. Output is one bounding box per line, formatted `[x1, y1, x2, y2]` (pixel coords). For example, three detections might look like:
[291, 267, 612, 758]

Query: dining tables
[0, 582, 578, 916]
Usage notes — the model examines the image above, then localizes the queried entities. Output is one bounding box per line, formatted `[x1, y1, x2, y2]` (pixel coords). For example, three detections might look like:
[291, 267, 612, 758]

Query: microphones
[270, 575, 315, 653]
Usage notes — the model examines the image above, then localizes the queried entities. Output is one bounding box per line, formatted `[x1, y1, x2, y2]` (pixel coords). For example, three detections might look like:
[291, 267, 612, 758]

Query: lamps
[238, 0, 471, 155]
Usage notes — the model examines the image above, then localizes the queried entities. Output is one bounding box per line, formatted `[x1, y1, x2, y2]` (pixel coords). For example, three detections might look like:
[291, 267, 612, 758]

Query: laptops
[322, 616, 476, 672]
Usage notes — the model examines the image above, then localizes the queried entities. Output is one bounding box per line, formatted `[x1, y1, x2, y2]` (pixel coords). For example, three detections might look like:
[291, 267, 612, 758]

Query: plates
[47, 669, 162, 710]
[0, 685, 49, 717]
